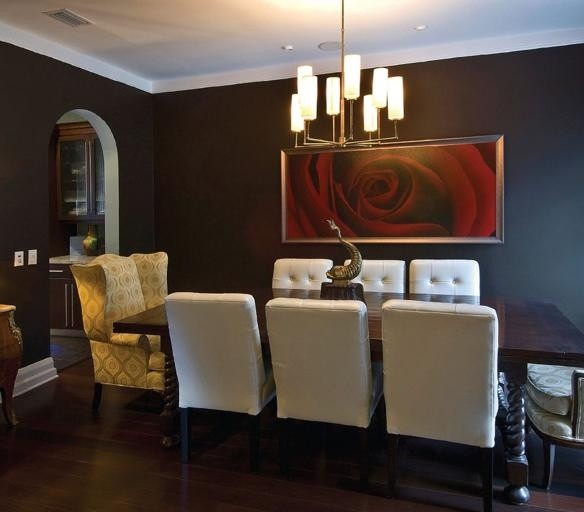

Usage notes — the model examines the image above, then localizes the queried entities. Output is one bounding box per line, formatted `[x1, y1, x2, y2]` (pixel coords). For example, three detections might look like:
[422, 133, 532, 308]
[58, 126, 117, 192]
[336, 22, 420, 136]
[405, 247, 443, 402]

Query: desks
[110, 291, 582, 507]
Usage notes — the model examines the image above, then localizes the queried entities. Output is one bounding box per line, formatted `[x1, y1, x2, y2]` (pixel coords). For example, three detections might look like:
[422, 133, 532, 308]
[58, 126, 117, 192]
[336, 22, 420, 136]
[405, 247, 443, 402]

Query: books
[63, 163, 104, 216]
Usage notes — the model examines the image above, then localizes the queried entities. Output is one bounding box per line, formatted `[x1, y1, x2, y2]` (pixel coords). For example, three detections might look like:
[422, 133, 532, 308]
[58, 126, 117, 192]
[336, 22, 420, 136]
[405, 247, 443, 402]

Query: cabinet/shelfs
[56, 122, 105, 224]
[50, 265, 87, 339]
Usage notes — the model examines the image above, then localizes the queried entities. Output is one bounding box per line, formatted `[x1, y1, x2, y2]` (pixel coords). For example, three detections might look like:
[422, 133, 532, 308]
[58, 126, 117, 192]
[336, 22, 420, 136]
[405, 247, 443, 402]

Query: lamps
[286, 1, 405, 148]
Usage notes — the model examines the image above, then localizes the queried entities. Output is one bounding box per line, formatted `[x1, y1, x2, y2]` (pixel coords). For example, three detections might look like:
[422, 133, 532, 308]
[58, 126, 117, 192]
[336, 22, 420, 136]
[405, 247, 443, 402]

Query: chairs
[382, 297, 501, 510]
[270, 257, 336, 290]
[164, 291, 276, 476]
[343, 258, 406, 292]
[526, 363, 583, 490]
[264, 295, 383, 492]
[407, 257, 483, 293]
[69, 251, 169, 410]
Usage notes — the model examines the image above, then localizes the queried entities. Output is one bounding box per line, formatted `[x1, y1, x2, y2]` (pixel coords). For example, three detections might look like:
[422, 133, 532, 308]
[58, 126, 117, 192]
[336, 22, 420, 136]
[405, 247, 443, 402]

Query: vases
[83, 224, 101, 255]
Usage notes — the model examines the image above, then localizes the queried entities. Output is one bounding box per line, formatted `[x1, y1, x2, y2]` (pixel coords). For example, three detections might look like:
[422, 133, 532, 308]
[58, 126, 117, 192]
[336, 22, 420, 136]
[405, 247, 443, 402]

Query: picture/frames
[280, 135, 504, 245]
